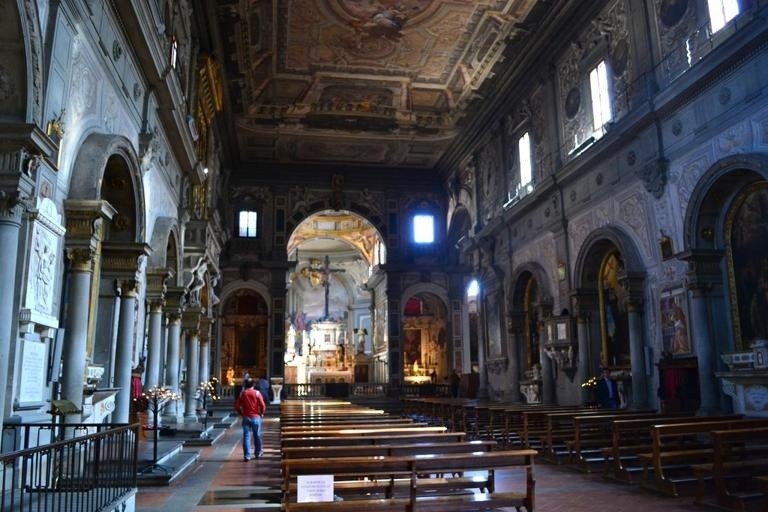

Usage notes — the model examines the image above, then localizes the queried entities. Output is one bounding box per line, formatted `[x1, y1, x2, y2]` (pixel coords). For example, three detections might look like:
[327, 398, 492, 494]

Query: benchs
[279, 395, 768, 512]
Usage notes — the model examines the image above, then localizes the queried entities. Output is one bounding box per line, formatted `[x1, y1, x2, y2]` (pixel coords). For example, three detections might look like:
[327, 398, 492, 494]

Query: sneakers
[245, 452, 264, 461]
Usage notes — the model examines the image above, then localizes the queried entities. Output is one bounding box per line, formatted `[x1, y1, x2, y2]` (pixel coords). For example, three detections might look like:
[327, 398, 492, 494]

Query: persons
[233, 378, 266, 462]
